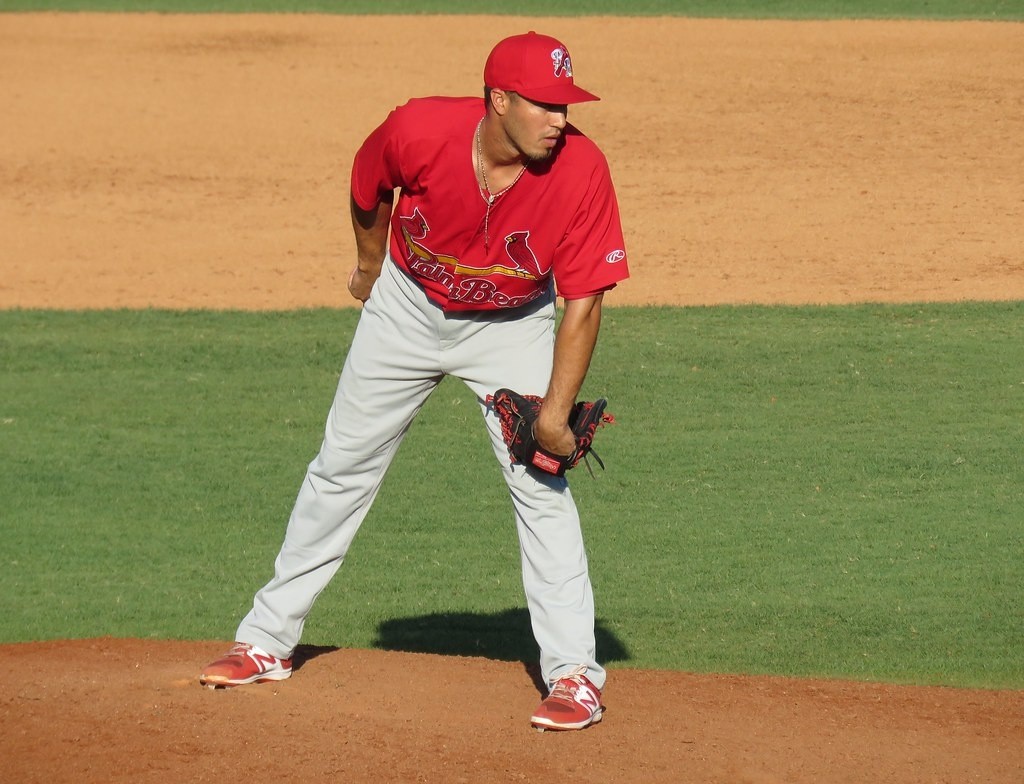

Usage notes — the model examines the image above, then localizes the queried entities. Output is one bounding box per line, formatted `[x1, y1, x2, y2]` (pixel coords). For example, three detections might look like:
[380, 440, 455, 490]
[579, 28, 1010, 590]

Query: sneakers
[530, 663, 602, 730]
[199, 641, 293, 686]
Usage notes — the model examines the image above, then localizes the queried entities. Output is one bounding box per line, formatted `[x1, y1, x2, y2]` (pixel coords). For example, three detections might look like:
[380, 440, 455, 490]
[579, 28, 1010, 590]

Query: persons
[200, 31, 631, 729]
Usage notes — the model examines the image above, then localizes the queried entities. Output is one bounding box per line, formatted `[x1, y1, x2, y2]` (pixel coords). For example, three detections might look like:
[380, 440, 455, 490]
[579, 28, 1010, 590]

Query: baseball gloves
[491, 387, 607, 483]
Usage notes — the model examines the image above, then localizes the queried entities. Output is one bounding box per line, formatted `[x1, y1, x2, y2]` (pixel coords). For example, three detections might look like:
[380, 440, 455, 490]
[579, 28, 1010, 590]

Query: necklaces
[475, 117, 531, 242]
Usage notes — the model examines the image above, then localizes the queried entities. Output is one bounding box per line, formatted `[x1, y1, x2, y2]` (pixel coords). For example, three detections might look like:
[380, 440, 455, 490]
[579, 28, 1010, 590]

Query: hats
[484, 31, 601, 104]
